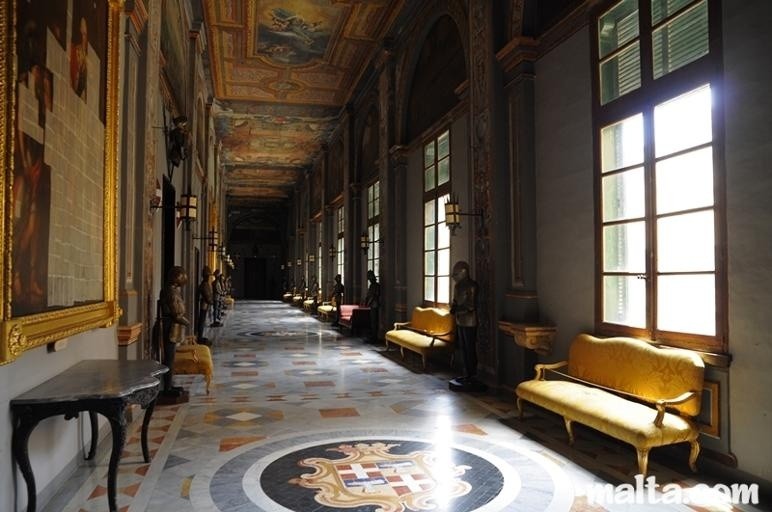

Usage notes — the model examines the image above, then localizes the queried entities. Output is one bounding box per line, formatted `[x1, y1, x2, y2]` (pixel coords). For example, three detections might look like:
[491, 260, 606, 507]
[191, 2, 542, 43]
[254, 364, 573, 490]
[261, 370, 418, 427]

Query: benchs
[385, 305, 458, 370]
[339, 303, 371, 335]
[283, 291, 337, 322]
[514, 332, 707, 485]
[172, 342, 213, 395]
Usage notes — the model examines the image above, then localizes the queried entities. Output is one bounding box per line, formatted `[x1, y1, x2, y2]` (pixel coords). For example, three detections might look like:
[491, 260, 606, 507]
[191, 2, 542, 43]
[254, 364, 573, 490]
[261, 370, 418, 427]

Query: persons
[279, 269, 381, 343]
[198, 266, 233, 342]
[72, 16, 89, 100]
[159, 266, 191, 398]
[450, 260, 479, 385]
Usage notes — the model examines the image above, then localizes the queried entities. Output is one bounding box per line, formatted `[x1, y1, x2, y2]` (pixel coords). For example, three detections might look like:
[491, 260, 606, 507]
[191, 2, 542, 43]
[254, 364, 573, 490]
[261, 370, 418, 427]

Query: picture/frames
[1, 1, 125, 364]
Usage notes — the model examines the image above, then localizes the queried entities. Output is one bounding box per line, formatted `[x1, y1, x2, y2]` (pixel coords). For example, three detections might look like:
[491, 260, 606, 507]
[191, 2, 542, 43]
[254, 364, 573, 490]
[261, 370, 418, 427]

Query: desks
[10, 359, 172, 511]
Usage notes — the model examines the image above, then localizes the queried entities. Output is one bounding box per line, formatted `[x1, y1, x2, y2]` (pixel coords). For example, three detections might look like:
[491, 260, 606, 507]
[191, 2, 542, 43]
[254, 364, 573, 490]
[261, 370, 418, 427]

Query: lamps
[445, 192, 484, 236]
[151, 193, 200, 232]
[192, 226, 227, 262]
[328, 243, 345, 263]
[359, 231, 385, 256]
